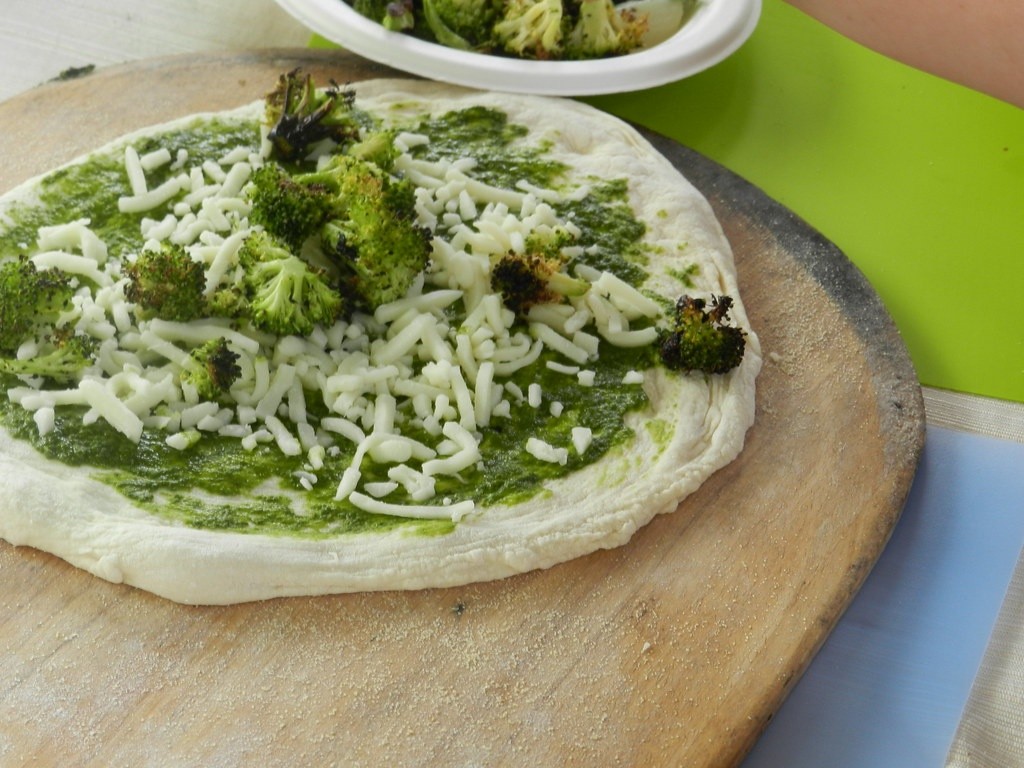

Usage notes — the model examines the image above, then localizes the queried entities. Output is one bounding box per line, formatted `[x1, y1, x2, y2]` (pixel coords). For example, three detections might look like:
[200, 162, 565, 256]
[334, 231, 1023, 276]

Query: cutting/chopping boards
[0, 51, 926, 767]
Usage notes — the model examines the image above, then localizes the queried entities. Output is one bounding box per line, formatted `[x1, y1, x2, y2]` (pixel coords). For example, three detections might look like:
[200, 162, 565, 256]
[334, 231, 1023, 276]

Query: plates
[274, 0, 761, 98]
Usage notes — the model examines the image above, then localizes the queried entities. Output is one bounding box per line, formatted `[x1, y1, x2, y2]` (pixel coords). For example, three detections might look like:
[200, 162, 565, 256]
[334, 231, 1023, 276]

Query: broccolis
[344, 1, 648, 60]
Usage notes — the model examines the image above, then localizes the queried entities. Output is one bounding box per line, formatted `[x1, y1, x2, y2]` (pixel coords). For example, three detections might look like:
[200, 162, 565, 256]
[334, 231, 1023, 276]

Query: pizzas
[1, 68, 762, 606]
